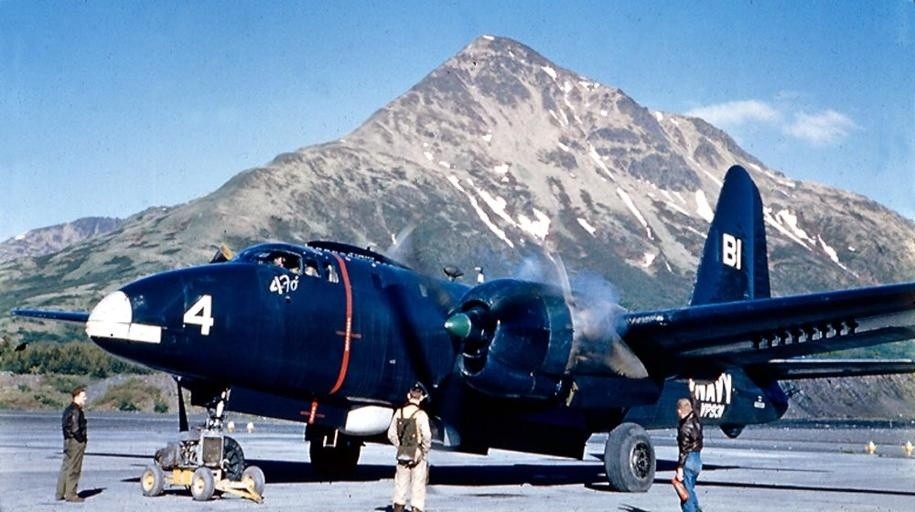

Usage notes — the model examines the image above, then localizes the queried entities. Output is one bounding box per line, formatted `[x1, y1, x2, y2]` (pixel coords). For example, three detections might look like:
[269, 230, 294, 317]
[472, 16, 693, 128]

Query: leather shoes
[55, 493, 84, 502]
[393, 504, 422, 512]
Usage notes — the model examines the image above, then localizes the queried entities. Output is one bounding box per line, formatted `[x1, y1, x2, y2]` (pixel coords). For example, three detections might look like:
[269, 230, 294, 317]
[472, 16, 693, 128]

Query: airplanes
[11, 166, 915, 494]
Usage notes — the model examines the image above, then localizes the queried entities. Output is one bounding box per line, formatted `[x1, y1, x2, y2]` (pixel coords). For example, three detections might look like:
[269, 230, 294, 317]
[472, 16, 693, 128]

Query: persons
[53, 385, 88, 503]
[257, 252, 287, 268]
[387, 386, 433, 511]
[675, 376, 703, 512]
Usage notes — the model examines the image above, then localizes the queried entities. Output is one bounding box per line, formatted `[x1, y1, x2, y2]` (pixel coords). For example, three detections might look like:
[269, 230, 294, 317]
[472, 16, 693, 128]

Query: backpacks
[397, 407, 420, 465]
